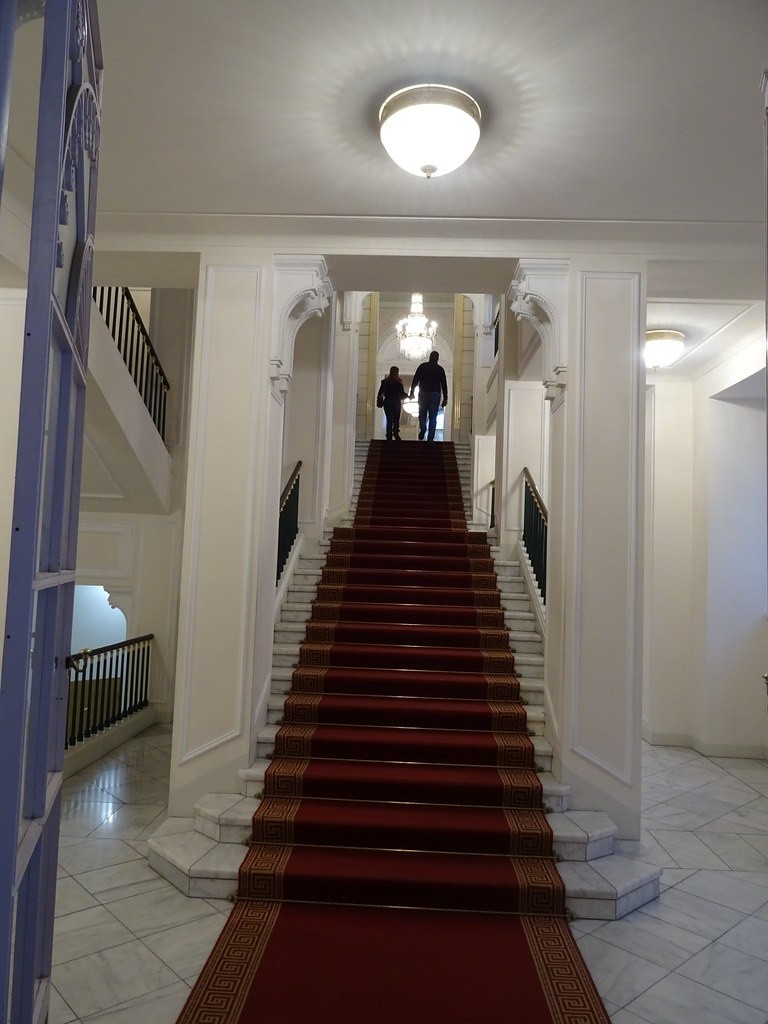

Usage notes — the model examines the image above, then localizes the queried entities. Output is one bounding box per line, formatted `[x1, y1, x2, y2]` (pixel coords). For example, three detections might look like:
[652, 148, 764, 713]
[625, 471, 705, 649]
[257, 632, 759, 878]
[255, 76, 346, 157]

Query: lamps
[377, 84, 481, 178]
[395, 290, 437, 360]
[402, 386, 420, 417]
[643, 330, 686, 371]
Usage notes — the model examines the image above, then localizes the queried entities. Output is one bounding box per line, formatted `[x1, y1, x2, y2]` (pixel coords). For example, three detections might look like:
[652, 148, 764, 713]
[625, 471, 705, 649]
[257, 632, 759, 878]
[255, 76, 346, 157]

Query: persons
[376, 366, 410, 440]
[410, 351, 448, 441]
